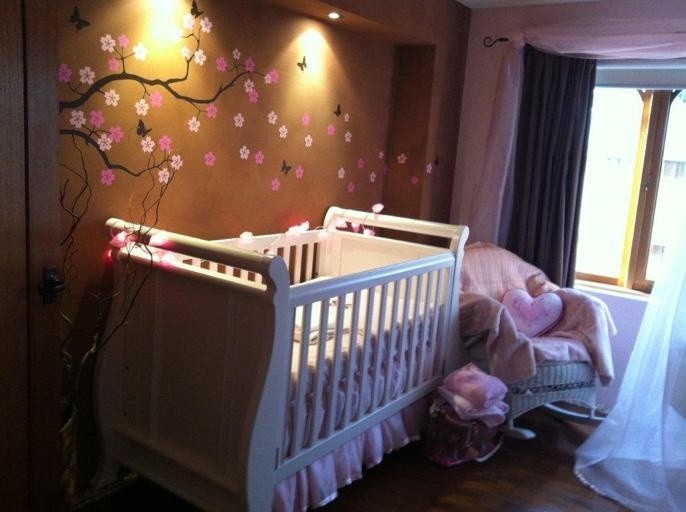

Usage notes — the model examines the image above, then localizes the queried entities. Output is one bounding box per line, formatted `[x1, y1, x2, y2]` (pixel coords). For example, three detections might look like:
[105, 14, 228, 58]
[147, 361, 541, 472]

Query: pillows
[501, 290, 563, 337]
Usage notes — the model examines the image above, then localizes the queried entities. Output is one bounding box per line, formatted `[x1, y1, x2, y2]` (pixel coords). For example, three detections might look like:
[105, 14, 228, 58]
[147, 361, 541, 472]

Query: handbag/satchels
[428, 397, 511, 466]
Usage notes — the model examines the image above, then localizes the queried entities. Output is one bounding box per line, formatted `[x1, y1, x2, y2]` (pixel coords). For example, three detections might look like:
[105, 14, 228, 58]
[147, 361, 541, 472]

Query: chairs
[458, 242, 610, 439]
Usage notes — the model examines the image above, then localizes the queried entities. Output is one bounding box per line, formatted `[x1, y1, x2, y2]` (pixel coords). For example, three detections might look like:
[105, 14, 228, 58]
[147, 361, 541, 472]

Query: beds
[86, 206, 470, 512]
